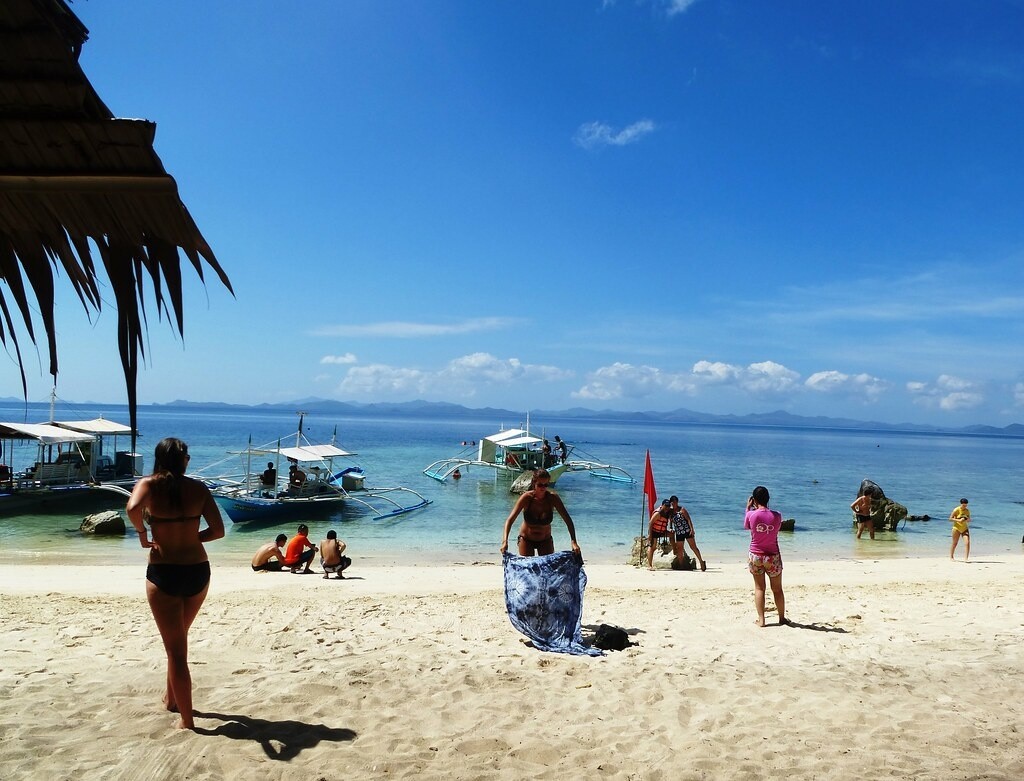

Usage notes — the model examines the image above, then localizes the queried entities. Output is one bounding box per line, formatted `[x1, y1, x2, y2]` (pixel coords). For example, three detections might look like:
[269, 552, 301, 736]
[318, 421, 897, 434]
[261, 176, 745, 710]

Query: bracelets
[136, 528, 147, 533]
[502, 540, 508, 544]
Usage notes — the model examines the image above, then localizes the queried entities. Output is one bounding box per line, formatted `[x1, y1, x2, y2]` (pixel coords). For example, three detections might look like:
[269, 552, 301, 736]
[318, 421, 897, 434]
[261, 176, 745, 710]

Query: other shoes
[701, 561, 706, 571]
[304, 569, 314, 574]
[290, 566, 296, 574]
[646, 566, 655, 571]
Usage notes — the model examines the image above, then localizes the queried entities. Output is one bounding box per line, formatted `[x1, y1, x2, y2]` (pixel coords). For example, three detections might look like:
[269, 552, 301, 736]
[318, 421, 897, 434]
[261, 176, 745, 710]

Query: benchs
[17, 462, 78, 489]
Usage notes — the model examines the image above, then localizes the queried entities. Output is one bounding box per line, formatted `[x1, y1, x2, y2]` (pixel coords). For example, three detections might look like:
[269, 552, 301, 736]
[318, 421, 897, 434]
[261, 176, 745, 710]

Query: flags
[643, 451, 658, 520]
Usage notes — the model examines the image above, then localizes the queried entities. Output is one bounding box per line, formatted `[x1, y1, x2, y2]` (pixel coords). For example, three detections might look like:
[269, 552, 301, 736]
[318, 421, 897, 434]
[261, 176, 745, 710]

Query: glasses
[184, 454, 191, 461]
[536, 483, 549, 488]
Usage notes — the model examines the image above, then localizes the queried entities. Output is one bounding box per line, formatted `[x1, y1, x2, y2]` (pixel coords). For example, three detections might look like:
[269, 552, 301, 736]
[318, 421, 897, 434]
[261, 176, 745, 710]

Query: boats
[0, 367, 144, 511]
[422, 410, 638, 487]
[185, 412, 433, 523]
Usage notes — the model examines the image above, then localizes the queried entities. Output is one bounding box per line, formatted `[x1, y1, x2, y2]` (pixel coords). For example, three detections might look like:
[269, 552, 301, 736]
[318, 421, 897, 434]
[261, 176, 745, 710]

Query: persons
[126, 438, 226, 728]
[851, 489, 875, 539]
[554, 435, 567, 463]
[320, 530, 351, 579]
[949, 498, 973, 563]
[542, 440, 552, 468]
[260, 462, 276, 486]
[499, 469, 580, 556]
[284, 525, 319, 573]
[252, 534, 303, 572]
[647, 495, 706, 571]
[288, 466, 308, 495]
[743, 486, 790, 626]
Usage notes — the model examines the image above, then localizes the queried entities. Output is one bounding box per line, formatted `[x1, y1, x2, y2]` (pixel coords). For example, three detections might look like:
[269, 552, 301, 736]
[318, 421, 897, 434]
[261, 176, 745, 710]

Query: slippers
[322, 576, 345, 579]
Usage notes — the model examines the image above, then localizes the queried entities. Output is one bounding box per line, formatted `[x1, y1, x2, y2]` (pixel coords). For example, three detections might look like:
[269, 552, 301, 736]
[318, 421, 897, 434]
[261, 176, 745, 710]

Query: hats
[960, 498, 969, 504]
[662, 499, 671, 507]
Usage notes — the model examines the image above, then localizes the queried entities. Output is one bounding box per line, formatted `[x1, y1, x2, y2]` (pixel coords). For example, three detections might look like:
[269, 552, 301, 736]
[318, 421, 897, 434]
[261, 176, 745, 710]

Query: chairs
[0, 466, 14, 493]
[287, 471, 319, 497]
[81, 466, 95, 482]
[246, 473, 283, 500]
